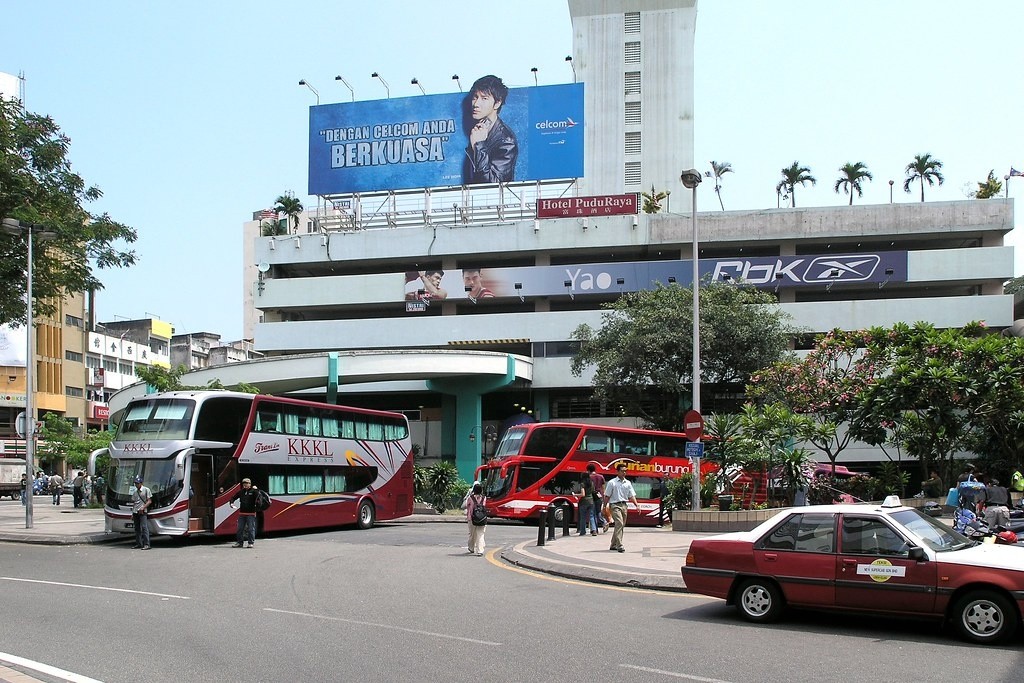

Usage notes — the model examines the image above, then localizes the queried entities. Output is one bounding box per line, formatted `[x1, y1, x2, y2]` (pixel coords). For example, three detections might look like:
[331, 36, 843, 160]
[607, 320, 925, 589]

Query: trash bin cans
[203, 516, 212, 530]
[718, 495, 734, 511]
[923, 505, 942, 516]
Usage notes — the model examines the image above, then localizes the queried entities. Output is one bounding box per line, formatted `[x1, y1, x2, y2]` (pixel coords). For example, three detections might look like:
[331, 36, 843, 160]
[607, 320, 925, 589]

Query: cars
[679, 494, 1024, 647]
[63, 480, 74, 495]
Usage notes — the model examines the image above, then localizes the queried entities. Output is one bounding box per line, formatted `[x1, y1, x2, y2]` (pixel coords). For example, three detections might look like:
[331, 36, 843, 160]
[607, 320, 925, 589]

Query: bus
[474, 421, 767, 527]
[84, 389, 416, 542]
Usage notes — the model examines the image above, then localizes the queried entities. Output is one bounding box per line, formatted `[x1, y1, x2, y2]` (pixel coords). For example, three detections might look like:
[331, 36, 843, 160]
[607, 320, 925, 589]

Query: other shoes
[618, 547, 625, 552]
[610, 547, 618, 550]
[232, 542, 243, 547]
[478, 551, 484, 555]
[132, 544, 143, 548]
[579, 533, 586, 536]
[247, 543, 254, 547]
[603, 522, 609, 532]
[913, 492, 924, 498]
[656, 524, 663, 528]
[590, 529, 598, 533]
[143, 545, 151, 550]
[468, 548, 474, 552]
[592, 531, 597, 535]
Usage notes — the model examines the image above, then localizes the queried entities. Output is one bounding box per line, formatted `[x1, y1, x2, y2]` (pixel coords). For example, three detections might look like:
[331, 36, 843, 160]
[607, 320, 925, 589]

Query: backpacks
[470, 494, 488, 526]
[256, 490, 272, 511]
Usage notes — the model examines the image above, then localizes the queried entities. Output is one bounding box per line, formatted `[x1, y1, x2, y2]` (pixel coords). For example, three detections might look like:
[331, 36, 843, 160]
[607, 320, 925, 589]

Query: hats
[134, 478, 143, 483]
[242, 478, 251, 483]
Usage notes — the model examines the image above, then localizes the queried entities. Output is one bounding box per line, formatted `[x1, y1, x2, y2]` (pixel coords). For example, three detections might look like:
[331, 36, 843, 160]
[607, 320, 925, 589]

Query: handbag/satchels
[147, 505, 152, 513]
[944, 487, 959, 507]
[594, 491, 603, 501]
[960, 473, 985, 495]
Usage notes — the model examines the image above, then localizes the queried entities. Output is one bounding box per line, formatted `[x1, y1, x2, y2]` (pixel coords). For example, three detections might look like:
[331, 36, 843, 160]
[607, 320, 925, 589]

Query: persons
[573, 472, 597, 536]
[601, 464, 641, 552]
[913, 463, 1024, 529]
[467, 484, 488, 556]
[229, 478, 263, 548]
[463, 75, 518, 185]
[656, 472, 673, 528]
[131, 477, 153, 550]
[585, 464, 609, 533]
[20, 471, 104, 508]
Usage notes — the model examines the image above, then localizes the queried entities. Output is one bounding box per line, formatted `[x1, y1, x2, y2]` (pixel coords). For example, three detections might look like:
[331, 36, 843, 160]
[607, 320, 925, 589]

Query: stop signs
[683, 409, 705, 441]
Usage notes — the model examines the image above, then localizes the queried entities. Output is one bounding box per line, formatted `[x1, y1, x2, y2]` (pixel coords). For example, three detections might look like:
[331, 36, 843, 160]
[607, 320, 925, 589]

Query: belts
[610, 501, 626, 505]
[988, 502, 1007, 506]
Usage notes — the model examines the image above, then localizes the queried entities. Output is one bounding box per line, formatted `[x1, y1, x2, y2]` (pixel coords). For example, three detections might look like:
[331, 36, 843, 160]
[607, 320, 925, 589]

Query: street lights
[469, 425, 498, 479]
[0, 217, 58, 527]
[680, 168, 703, 511]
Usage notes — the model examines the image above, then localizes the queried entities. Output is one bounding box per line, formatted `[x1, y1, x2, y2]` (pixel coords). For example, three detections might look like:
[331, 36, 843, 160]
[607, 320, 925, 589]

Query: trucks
[0, 457, 26, 500]
